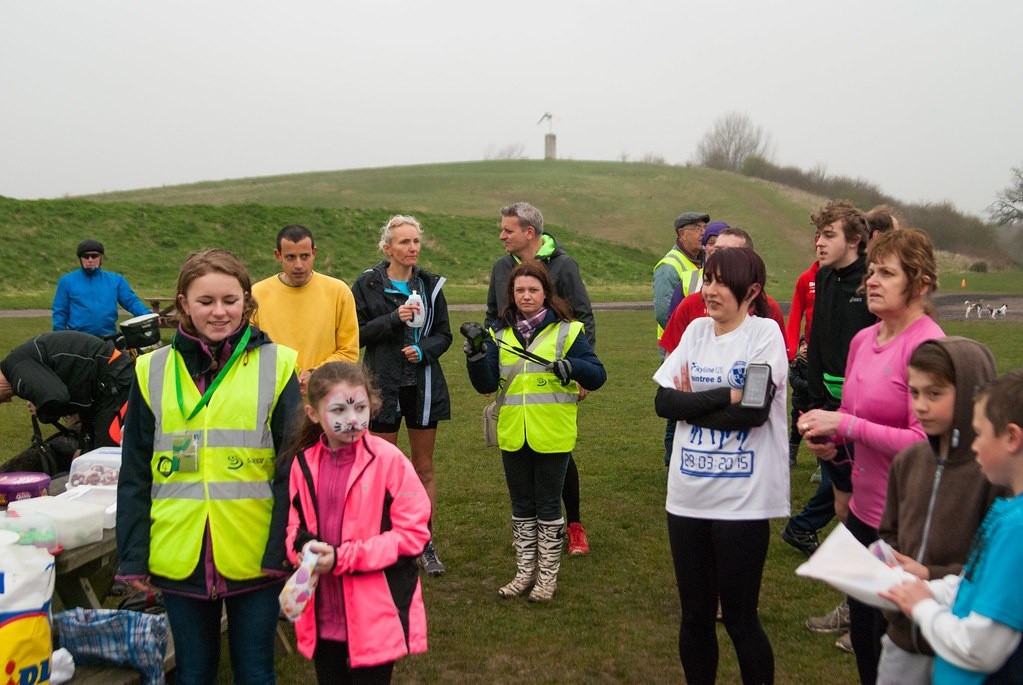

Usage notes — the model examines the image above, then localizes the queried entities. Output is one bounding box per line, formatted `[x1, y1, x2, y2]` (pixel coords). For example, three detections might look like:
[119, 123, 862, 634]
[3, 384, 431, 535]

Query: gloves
[464, 338, 488, 361]
[544, 359, 571, 379]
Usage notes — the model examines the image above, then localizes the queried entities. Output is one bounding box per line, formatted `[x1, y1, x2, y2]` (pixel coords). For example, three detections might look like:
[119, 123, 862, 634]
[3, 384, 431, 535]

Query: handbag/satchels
[483, 401, 499, 447]
[823, 373, 845, 399]
[0, 413, 85, 478]
[52, 606, 170, 685]
[278, 540, 320, 620]
[118, 589, 168, 616]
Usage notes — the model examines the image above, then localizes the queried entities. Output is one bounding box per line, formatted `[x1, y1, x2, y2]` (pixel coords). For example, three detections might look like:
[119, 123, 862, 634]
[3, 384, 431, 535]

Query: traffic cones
[961, 278, 966, 288]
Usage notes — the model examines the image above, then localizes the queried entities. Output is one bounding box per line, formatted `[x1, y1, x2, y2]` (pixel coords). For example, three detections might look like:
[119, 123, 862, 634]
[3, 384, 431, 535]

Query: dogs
[965, 298, 983, 319]
[987, 303, 1008, 319]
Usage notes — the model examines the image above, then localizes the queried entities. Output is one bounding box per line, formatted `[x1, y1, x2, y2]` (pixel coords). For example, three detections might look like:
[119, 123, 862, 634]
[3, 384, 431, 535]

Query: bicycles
[100, 301, 174, 360]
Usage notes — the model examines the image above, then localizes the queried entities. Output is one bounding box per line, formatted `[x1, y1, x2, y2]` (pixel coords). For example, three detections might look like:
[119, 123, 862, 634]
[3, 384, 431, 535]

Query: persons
[0, 330, 136, 456]
[285, 361, 433, 685]
[870, 336, 997, 685]
[481, 202, 597, 556]
[655, 220, 785, 620]
[351, 215, 453, 573]
[52, 239, 161, 350]
[797, 227, 947, 685]
[116, 247, 306, 685]
[885, 368, 1023, 684]
[652, 246, 791, 685]
[460, 262, 608, 602]
[786, 199, 902, 558]
[248, 225, 361, 621]
[653, 212, 710, 367]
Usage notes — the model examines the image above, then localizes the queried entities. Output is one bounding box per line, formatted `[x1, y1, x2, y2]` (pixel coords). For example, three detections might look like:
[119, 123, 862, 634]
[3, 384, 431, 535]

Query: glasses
[683, 225, 709, 233]
[79, 252, 101, 259]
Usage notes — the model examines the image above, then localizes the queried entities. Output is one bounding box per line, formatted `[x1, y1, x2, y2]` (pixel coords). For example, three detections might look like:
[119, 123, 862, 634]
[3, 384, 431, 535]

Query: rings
[803, 423, 809, 430]
[808, 431, 812, 436]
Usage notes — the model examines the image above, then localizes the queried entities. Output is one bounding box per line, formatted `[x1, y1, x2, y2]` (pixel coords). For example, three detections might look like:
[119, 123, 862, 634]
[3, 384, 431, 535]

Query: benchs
[65, 597, 292, 685]
[159, 314, 176, 318]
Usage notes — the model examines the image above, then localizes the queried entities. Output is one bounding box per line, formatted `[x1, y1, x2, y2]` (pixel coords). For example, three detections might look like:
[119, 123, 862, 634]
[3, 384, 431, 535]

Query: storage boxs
[120, 313, 161, 349]
[6, 447, 122, 550]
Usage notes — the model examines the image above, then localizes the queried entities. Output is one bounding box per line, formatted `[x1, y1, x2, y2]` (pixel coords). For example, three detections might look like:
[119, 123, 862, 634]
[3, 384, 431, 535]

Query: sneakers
[805, 600, 850, 633]
[416, 541, 446, 574]
[835, 630, 854, 653]
[781, 516, 820, 558]
[566, 522, 590, 555]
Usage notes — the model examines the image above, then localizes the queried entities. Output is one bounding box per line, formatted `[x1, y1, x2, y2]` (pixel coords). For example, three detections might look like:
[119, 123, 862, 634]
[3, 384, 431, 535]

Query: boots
[789, 441, 799, 467]
[497, 515, 538, 597]
[529, 516, 565, 601]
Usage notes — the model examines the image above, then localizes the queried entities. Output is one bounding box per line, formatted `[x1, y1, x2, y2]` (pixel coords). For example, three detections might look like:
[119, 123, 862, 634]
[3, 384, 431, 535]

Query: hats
[674, 212, 710, 234]
[76, 240, 105, 258]
[702, 221, 729, 246]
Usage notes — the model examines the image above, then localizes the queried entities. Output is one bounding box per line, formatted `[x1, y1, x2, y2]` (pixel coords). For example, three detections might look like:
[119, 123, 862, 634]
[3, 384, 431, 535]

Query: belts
[105, 357, 134, 381]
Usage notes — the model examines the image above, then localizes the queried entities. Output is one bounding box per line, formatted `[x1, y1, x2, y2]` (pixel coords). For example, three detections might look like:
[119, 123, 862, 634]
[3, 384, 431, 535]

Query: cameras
[460, 322, 485, 353]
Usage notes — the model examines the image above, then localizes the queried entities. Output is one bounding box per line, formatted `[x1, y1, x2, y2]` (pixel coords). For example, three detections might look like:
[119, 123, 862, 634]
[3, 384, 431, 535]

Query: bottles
[403, 290, 425, 327]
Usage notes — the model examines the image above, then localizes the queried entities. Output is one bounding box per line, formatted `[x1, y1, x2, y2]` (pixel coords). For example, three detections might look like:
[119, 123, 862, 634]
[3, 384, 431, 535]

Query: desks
[145, 298, 176, 314]
[0, 470, 118, 610]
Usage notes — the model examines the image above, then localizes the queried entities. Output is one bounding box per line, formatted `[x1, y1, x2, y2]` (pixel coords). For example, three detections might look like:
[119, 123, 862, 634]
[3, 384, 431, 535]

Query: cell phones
[741, 364, 770, 409]
